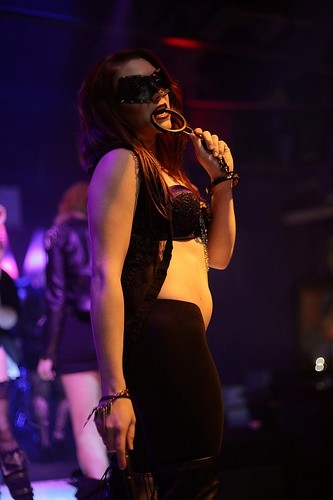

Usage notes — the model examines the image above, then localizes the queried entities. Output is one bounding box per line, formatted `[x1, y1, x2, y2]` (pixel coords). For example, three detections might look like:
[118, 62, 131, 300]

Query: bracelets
[83, 388, 130, 433]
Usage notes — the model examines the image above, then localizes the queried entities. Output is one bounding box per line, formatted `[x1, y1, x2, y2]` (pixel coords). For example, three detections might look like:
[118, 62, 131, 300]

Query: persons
[69, 48, 240, 500]
[0, 181, 112, 500]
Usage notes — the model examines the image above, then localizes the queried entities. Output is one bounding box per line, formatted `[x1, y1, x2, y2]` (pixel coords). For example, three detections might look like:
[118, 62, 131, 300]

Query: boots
[159, 457, 223, 499]
[108, 460, 159, 500]
[0, 448, 35, 500]
